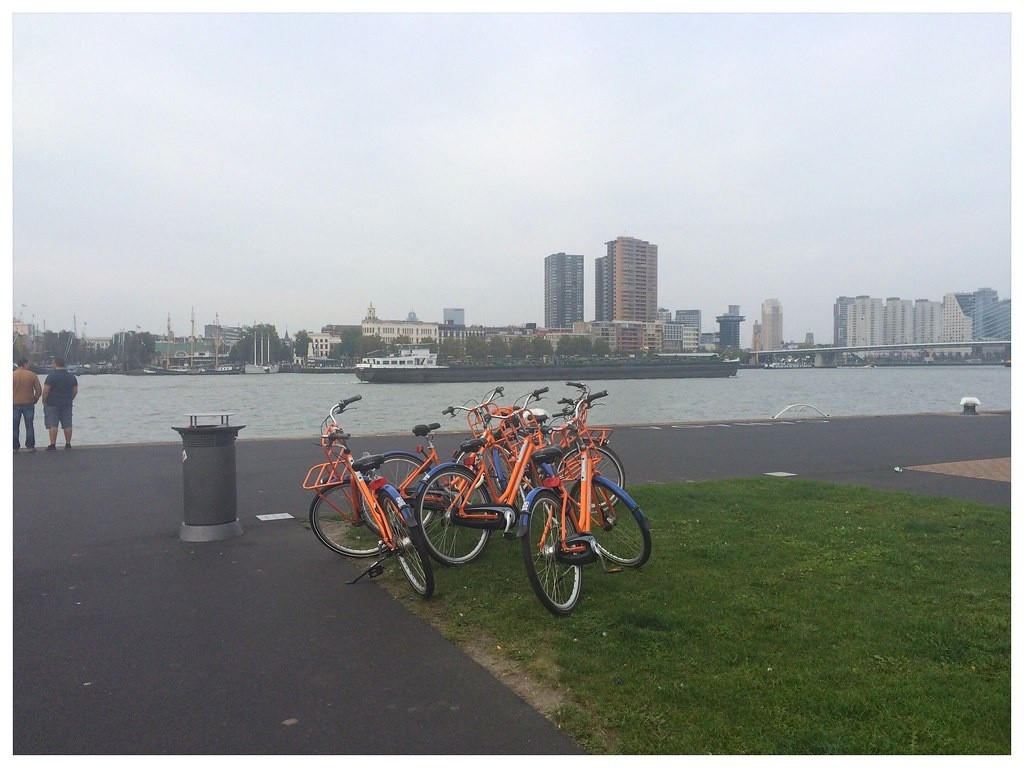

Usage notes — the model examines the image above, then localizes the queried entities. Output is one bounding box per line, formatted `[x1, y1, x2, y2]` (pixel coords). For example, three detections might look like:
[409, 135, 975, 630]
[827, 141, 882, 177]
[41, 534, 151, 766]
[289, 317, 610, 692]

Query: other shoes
[27, 446, 34, 449]
[66, 443, 71, 448]
[48, 444, 55, 447]
[13, 448, 20, 452]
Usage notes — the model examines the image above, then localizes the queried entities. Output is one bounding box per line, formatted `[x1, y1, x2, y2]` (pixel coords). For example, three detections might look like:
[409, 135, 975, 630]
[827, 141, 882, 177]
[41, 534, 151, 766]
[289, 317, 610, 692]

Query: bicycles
[358, 382, 651, 618]
[302, 395, 434, 599]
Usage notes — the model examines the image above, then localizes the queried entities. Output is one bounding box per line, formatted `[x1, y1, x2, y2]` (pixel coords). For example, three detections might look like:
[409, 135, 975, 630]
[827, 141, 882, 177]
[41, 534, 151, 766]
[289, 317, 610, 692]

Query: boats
[245, 365, 280, 374]
[354, 343, 740, 383]
[143, 368, 245, 375]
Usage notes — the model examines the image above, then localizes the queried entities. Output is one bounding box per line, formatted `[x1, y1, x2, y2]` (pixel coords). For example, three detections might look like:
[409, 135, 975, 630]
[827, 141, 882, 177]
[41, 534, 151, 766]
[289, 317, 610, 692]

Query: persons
[42, 357, 78, 448]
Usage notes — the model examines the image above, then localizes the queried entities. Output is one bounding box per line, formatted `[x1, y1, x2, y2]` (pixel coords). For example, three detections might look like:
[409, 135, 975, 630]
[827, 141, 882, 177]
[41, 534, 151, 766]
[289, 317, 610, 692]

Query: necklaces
[13, 358, 42, 452]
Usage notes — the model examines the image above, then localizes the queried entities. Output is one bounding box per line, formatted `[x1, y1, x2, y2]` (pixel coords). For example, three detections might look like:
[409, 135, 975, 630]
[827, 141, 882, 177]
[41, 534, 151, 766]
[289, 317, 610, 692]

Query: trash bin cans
[170, 412, 246, 529]
